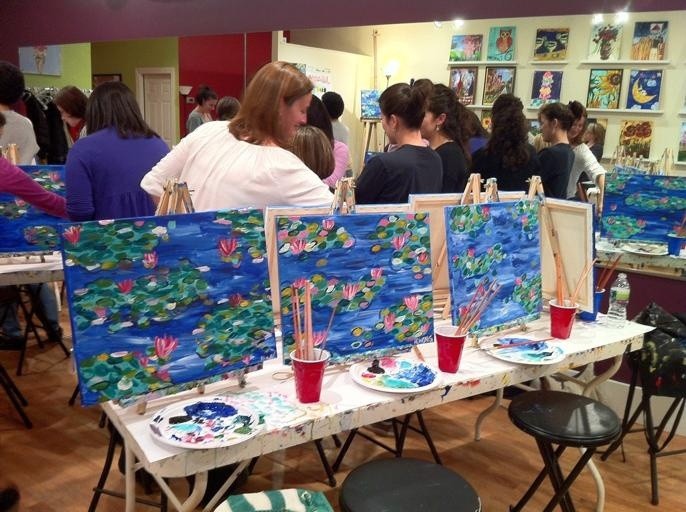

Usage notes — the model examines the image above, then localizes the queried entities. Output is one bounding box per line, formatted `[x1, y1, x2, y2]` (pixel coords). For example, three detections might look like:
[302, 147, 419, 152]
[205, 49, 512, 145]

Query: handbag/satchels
[627, 299, 686, 395]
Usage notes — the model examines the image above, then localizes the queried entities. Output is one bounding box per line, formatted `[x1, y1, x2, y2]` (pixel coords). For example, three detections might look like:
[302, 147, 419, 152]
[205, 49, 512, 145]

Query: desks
[2, 255, 66, 285]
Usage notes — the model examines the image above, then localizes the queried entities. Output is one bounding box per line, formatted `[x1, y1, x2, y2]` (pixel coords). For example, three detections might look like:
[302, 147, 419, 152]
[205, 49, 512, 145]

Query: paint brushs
[456, 278, 502, 336]
[678, 215, 685, 237]
[597, 254, 623, 291]
[553, 249, 598, 306]
[290, 278, 338, 360]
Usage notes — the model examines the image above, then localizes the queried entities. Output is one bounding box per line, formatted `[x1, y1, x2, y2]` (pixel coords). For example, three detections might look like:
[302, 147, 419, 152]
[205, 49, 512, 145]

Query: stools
[2, 282, 79, 429]
[88, 300, 685, 511]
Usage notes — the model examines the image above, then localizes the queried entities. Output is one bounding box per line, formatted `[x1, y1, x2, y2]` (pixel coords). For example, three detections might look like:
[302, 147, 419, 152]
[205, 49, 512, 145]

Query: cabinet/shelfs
[446, 58, 521, 112]
[575, 57, 666, 165]
[525, 60, 568, 112]
[673, 108, 686, 170]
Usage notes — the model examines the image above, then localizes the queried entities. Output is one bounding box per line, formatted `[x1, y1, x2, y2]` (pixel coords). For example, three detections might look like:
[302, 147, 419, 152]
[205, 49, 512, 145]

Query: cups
[434, 326, 471, 374]
[548, 299, 580, 340]
[595, 287, 606, 312]
[289, 349, 331, 404]
[667, 233, 686, 256]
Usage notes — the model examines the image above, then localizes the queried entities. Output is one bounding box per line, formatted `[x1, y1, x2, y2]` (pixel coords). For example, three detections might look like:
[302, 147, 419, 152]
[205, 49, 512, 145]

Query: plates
[149, 395, 269, 450]
[622, 243, 669, 256]
[478, 334, 567, 366]
[348, 356, 443, 393]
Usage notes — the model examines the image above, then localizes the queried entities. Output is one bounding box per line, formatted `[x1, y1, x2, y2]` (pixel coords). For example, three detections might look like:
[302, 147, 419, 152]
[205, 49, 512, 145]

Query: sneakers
[0, 484, 23, 512]
[1, 334, 25, 350]
[363, 417, 398, 438]
[46, 324, 63, 342]
[187, 461, 249, 510]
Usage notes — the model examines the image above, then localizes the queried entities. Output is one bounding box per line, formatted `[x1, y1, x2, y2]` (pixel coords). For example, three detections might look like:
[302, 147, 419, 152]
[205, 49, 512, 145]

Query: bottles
[605, 272, 631, 329]
